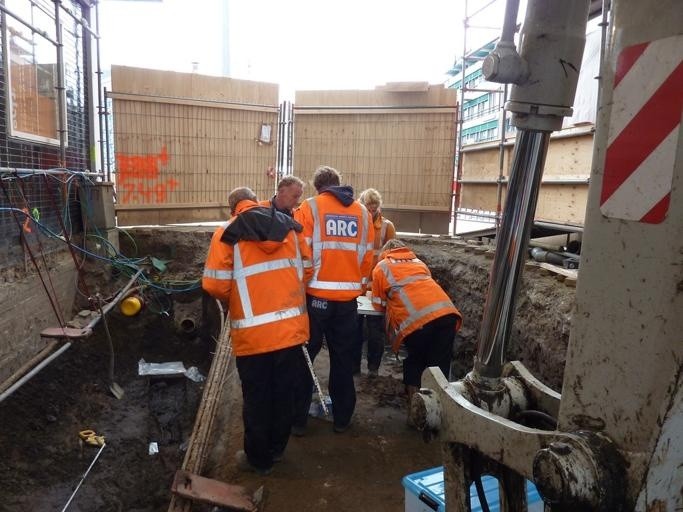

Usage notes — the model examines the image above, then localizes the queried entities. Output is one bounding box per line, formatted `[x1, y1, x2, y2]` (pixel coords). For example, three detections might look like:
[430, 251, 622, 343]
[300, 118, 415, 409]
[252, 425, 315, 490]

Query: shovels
[95, 293, 124, 401]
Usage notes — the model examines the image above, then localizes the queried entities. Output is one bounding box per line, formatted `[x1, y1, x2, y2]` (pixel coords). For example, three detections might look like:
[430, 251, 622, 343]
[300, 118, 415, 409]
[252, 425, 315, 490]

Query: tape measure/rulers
[79, 429, 106, 448]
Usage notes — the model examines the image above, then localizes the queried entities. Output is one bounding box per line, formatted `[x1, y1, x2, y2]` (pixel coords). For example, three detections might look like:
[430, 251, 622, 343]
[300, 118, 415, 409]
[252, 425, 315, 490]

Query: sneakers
[294, 428, 305, 436]
[237, 449, 281, 474]
[334, 417, 352, 432]
[367, 369, 378, 378]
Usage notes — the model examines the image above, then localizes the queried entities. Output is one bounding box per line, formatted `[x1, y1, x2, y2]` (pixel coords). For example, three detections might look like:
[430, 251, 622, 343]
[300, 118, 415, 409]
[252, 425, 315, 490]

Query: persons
[258, 177, 304, 217]
[291, 164, 375, 435]
[370, 238, 464, 433]
[202, 186, 312, 475]
[349, 188, 396, 376]
